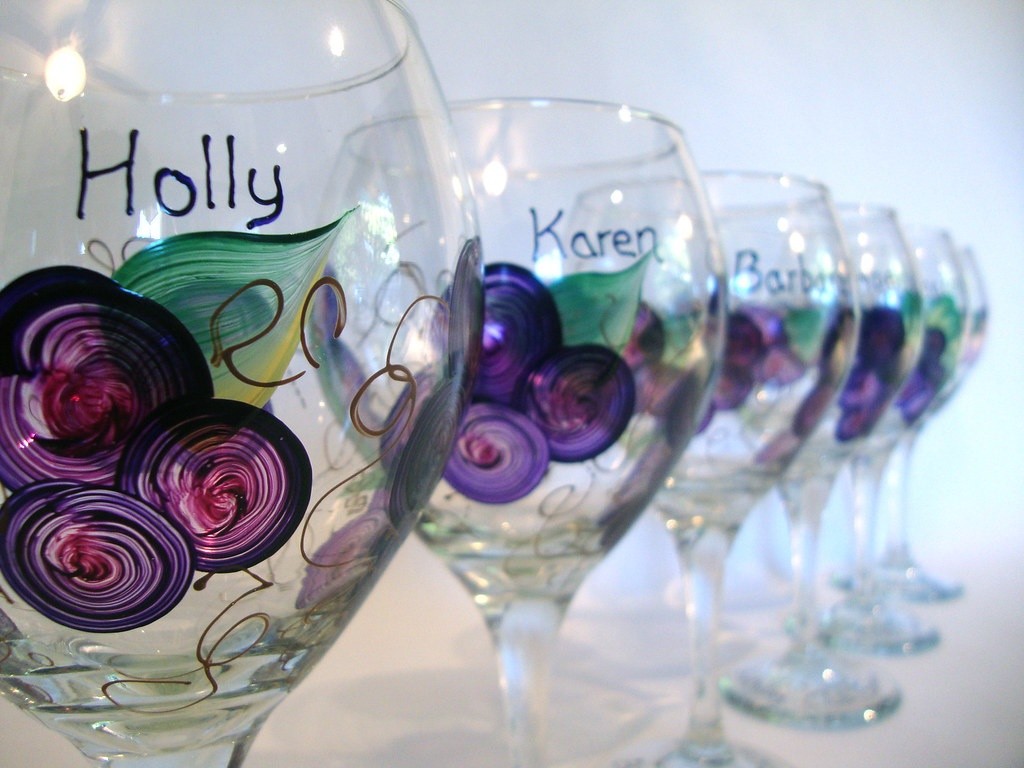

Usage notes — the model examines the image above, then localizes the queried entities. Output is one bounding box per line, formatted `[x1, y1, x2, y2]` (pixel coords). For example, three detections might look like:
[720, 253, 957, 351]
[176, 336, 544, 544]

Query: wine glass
[720, 195, 991, 727]
[304, 92, 729, 768]
[561, 165, 858, 768]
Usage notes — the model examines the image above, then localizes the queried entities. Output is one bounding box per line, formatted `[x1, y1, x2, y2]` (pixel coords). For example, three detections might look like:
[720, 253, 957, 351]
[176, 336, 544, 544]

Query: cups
[0, 0, 484, 768]
